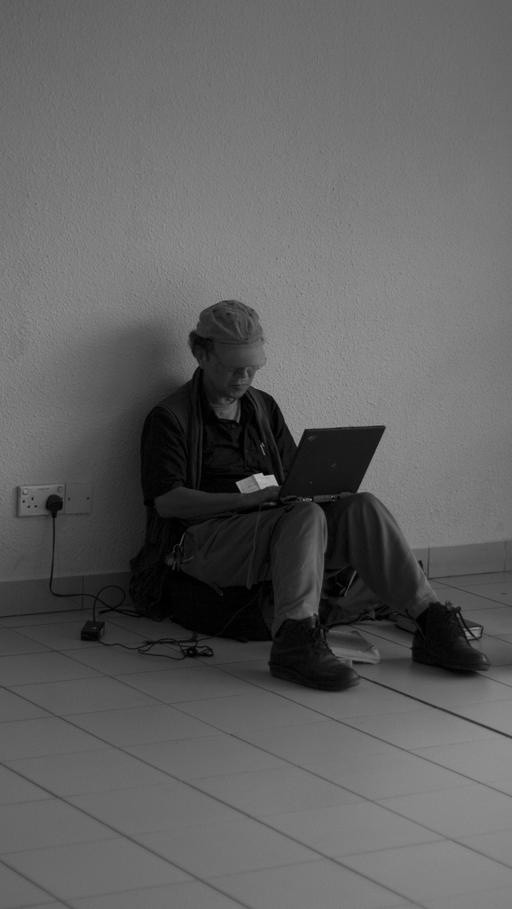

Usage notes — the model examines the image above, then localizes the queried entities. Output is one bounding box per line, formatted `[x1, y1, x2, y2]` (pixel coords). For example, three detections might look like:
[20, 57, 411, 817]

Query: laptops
[254, 424, 388, 504]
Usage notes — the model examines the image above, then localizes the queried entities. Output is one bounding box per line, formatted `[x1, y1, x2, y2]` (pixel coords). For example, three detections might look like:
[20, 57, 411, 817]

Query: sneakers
[412, 603, 491, 672]
[268, 617, 359, 691]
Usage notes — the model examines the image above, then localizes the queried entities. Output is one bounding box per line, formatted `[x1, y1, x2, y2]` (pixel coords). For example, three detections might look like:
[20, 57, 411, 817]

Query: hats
[195, 300, 267, 370]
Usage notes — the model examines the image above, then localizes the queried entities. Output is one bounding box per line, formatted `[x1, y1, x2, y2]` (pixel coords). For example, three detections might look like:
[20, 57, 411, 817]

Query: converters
[82, 618, 106, 643]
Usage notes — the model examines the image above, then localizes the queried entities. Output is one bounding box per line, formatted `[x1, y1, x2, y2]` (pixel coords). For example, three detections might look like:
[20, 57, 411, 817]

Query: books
[393, 609, 485, 641]
[323, 626, 381, 668]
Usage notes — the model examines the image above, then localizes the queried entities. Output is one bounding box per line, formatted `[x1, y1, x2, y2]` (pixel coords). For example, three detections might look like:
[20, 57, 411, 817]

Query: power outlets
[17, 483, 64, 517]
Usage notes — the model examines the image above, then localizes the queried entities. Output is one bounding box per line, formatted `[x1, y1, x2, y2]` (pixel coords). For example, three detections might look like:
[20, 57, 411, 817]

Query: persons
[126, 300, 492, 690]
